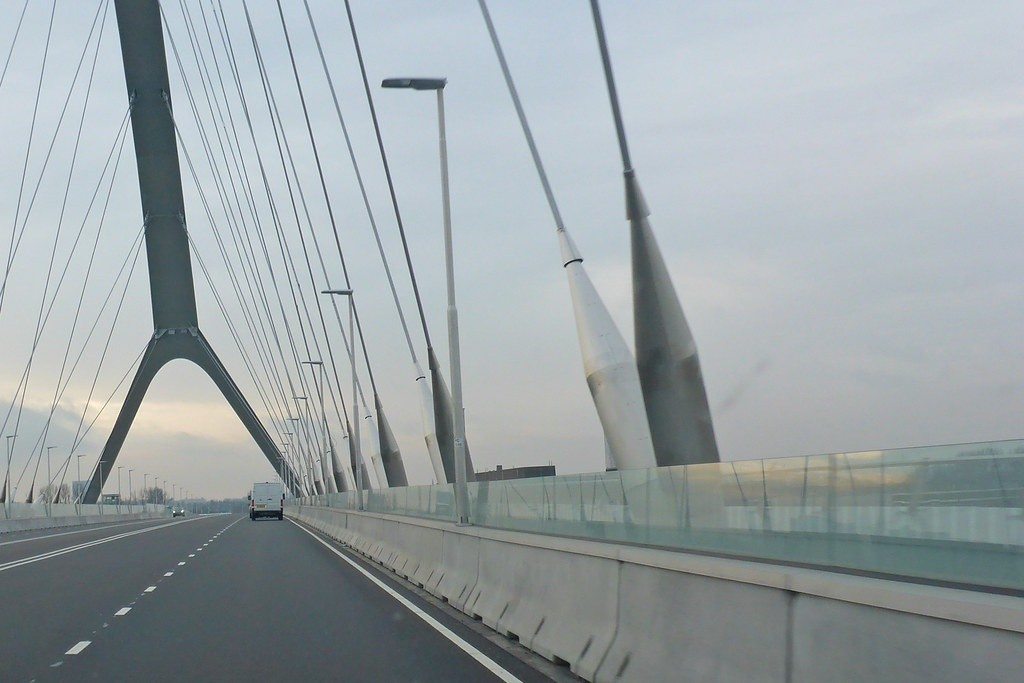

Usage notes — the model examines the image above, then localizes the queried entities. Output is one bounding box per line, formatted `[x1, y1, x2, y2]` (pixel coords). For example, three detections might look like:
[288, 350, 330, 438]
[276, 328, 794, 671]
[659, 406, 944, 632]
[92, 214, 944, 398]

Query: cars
[173, 506, 185, 518]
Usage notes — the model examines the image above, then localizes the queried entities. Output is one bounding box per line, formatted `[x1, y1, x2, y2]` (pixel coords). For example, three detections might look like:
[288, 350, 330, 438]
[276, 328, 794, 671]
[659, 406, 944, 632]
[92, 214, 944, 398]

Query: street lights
[292, 396, 312, 506]
[380, 77, 473, 527]
[129, 469, 134, 514]
[144, 473, 149, 514]
[287, 418, 304, 506]
[277, 432, 294, 503]
[100, 461, 109, 515]
[47, 446, 57, 517]
[321, 289, 364, 511]
[7, 434, 19, 518]
[155, 476, 193, 512]
[118, 466, 125, 514]
[77, 454, 86, 516]
[302, 361, 333, 506]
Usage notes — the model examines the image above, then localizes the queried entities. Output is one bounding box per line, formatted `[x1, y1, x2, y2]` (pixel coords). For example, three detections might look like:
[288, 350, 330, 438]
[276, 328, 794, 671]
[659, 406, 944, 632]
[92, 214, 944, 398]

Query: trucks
[248, 482, 285, 520]
